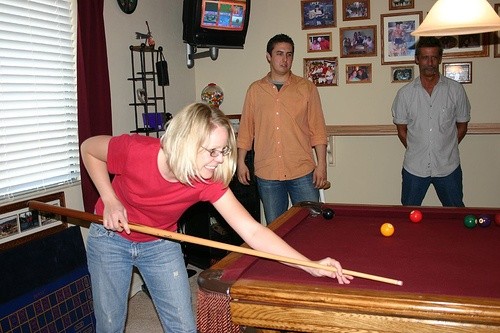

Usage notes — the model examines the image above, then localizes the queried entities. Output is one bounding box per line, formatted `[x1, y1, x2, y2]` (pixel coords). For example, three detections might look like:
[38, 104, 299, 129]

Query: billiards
[464, 214, 491, 228]
[322, 208, 335, 220]
[381, 222, 395, 237]
[410, 210, 423, 223]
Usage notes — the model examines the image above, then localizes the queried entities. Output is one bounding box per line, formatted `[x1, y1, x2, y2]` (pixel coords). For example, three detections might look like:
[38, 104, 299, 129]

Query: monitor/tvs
[182, 0, 251, 50]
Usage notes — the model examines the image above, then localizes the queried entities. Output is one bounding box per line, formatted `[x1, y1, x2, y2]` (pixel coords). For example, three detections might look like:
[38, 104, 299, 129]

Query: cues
[27, 199, 403, 286]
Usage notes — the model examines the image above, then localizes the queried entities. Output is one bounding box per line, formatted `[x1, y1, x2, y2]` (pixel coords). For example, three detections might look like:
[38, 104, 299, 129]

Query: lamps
[410, 0, 500, 36]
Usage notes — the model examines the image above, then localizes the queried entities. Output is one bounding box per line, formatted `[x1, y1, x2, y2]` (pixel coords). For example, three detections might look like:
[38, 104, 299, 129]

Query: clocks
[117, 0, 138, 14]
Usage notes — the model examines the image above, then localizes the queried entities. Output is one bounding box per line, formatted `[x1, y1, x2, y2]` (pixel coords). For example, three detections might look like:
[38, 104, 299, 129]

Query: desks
[195, 201, 500, 333]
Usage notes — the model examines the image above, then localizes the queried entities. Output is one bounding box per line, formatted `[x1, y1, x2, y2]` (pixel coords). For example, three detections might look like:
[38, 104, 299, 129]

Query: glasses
[201, 146, 232, 157]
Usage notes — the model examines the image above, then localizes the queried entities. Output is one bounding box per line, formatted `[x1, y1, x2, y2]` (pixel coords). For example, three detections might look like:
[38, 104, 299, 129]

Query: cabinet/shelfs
[128, 44, 173, 139]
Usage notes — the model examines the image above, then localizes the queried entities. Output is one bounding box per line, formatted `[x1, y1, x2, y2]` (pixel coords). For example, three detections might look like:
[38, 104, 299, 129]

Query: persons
[236, 33, 328, 225]
[80, 103, 354, 333]
[391, 36, 472, 208]
[304, 0, 481, 88]
[0, 204, 60, 238]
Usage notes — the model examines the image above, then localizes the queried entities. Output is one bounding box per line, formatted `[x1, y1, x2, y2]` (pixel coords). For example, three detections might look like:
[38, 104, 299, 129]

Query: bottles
[201, 83, 224, 106]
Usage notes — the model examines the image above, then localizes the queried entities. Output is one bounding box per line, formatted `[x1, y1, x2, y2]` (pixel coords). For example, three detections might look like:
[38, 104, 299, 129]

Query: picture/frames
[300, 0, 500, 87]
[0, 190, 68, 251]
